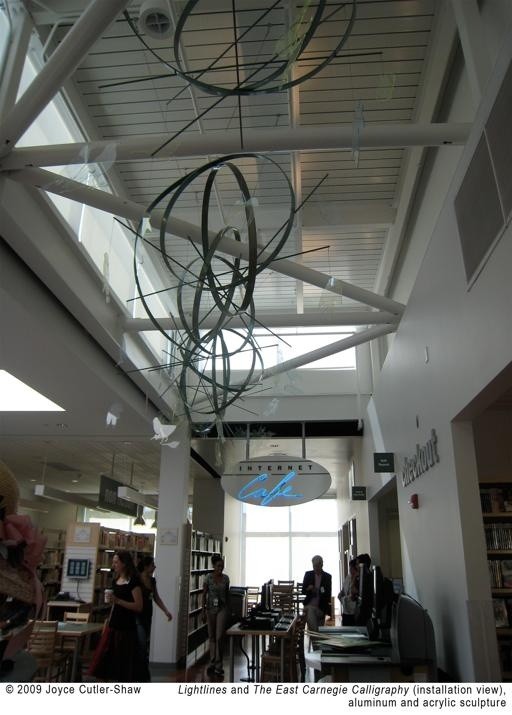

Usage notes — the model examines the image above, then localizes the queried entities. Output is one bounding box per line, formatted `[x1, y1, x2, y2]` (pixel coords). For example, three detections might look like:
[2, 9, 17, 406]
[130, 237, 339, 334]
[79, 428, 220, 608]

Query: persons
[337, 553, 394, 647]
[201, 556, 230, 674]
[301, 555, 332, 651]
[136, 555, 172, 669]
[96, 549, 149, 682]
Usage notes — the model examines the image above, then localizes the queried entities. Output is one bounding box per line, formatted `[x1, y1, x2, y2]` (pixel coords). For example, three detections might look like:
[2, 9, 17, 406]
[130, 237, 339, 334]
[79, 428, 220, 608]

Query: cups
[105, 590, 113, 603]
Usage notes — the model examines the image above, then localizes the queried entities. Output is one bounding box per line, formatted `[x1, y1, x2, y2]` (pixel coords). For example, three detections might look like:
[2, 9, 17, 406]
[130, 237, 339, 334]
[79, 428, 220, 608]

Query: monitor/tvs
[369, 565, 393, 631]
[357, 564, 370, 607]
[66, 558, 91, 579]
[250, 578, 276, 620]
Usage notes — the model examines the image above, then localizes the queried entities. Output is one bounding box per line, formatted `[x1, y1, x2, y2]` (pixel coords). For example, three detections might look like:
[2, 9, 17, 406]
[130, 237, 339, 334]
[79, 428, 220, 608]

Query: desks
[317, 626, 458, 682]
[226, 613, 298, 683]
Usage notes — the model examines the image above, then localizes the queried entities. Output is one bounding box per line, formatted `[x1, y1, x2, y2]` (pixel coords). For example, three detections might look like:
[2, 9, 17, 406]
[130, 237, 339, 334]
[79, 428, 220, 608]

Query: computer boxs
[247, 608, 283, 630]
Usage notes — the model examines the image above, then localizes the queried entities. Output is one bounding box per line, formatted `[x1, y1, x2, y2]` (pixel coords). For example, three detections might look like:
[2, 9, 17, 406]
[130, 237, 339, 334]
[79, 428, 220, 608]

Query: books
[95, 531, 153, 589]
[479, 489, 512, 628]
[304, 625, 384, 656]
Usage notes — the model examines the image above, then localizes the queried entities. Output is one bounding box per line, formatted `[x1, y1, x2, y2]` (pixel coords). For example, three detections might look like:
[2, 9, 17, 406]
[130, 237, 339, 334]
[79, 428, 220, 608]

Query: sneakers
[207, 665, 225, 675]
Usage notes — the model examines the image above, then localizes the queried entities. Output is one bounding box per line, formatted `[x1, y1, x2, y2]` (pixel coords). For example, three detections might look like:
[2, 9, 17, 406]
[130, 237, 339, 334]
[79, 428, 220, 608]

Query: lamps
[34, 456, 111, 513]
[117, 461, 159, 510]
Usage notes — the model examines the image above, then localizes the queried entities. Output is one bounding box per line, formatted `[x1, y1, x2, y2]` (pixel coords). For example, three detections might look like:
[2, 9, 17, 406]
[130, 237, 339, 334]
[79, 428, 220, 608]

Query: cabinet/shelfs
[37, 521, 223, 671]
[478, 482, 512, 682]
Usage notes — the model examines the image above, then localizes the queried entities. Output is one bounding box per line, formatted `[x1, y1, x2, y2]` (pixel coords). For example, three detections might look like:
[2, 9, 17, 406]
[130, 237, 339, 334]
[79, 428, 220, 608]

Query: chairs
[28, 602, 110, 682]
[246, 580, 309, 683]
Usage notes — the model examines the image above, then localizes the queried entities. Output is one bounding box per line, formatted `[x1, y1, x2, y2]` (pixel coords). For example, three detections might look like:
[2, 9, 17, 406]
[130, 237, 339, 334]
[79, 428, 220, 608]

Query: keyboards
[275, 612, 295, 631]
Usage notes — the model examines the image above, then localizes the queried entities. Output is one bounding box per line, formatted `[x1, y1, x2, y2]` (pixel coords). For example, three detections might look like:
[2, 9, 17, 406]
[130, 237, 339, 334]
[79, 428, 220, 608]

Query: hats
[0, 457, 40, 640]
